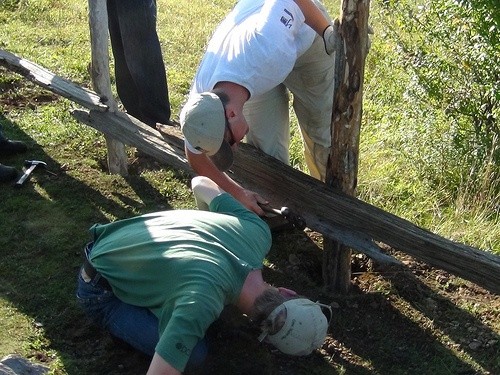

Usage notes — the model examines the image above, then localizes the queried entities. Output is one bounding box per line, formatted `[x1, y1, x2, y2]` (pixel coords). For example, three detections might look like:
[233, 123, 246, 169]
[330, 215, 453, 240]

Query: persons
[76, 175, 332, 375]
[107, 0, 171, 159]
[179, 0, 374, 272]
[0, 124, 27, 181]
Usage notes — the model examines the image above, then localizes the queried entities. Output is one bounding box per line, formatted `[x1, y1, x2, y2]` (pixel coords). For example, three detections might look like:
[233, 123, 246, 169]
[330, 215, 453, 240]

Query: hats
[257, 299, 329, 356]
[180, 92, 234, 173]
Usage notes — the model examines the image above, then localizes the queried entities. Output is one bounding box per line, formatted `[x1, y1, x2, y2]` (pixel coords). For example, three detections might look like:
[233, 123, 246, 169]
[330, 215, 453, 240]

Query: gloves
[322, 16, 375, 61]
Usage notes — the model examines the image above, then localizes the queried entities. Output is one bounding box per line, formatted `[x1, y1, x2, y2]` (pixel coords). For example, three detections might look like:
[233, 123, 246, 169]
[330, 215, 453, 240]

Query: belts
[83, 240, 113, 294]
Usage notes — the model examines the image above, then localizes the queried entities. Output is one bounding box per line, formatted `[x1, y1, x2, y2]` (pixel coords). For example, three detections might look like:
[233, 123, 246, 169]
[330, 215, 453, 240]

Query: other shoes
[0, 139, 27, 154]
[256, 211, 303, 232]
[0, 163, 20, 184]
[129, 119, 181, 165]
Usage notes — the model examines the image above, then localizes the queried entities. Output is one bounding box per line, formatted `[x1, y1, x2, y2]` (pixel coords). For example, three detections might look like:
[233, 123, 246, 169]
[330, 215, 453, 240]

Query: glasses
[316, 302, 332, 327]
[223, 103, 236, 146]
